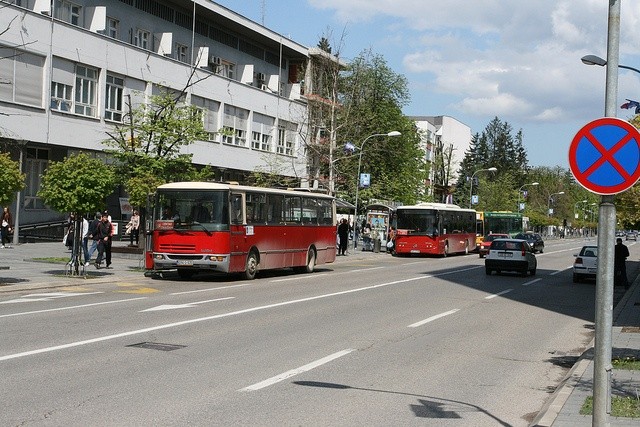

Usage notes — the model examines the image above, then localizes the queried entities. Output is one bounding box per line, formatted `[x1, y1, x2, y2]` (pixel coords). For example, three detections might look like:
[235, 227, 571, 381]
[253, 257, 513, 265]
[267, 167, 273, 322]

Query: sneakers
[1, 245, 5, 249]
[130, 244, 132, 246]
[94, 263, 100, 268]
[106, 264, 113, 268]
[85, 261, 90, 266]
[135, 244, 138, 246]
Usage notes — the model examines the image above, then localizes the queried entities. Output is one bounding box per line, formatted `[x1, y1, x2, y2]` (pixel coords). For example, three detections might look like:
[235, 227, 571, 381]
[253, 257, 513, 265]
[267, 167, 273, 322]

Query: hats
[95, 212, 101, 217]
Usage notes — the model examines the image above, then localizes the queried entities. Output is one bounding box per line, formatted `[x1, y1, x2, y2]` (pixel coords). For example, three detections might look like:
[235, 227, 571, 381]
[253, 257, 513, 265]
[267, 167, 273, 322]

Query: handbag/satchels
[66, 227, 74, 246]
[361, 234, 369, 238]
[387, 238, 394, 248]
[126, 226, 132, 235]
[63, 227, 74, 250]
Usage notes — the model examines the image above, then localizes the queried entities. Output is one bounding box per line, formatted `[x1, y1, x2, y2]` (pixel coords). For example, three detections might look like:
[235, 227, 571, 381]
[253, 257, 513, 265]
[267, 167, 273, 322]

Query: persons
[105, 211, 112, 224]
[71, 213, 90, 266]
[161, 204, 181, 224]
[336, 219, 349, 256]
[129, 210, 140, 246]
[68, 211, 79, 266]
[335, 219, 344, 251]
[335, 221, 339, 228]
[234, 198, 241, 220]
[94, 214, 115, 269]
[363, 175, 369, 188]
[614, 237, 630, 285]
[351, 221, 379, 251]
[389, 225, 395, 253]
[187, 198, 212, 223]
[94, 212, 100, 221]
[1, 207, 13, 249]
[83, 213, 105, 264]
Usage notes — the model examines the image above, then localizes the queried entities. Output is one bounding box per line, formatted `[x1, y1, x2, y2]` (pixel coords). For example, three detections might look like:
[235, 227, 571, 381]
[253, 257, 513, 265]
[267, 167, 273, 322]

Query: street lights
[547, 191, 565, 217]
[517, 182, 540, 213]
[583, 203, 596, 221]
[352, 130, 402, 251]
[574, 200, 588, 221]
[469, 167, 497, 209]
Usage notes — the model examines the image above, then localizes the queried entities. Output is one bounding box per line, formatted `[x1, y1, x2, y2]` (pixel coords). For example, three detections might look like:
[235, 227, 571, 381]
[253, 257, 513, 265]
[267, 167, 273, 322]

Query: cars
[479, 233, 512, 258]
[515, 232, 544, 254]
[573, 246, 598, 283]
[626, 233, 636, 241]
[616, 232, 624, 236]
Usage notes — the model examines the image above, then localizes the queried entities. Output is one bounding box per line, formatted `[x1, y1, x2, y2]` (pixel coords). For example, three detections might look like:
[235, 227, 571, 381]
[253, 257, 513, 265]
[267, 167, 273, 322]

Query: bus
[389, 202, 477, 257]
[145, 181, 336, 279]
[476, 210, 529, 251]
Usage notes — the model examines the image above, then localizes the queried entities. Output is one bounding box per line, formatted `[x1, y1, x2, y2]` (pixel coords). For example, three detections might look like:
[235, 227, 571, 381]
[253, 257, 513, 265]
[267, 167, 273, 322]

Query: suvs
[485, 239, 536, 277]
